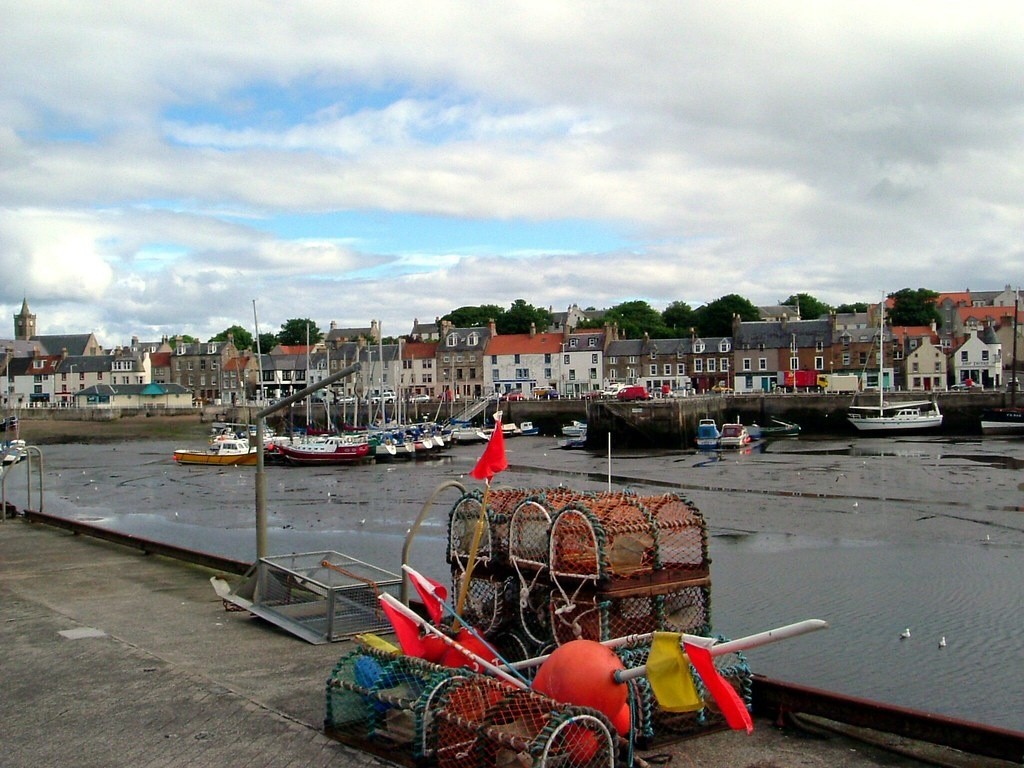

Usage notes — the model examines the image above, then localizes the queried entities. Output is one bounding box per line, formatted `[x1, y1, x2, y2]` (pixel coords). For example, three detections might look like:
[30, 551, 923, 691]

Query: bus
[532, 386, 557, 397]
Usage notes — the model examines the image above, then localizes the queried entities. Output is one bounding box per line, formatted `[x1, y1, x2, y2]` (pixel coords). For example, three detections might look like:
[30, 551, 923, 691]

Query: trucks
[601, 385, 632, 399]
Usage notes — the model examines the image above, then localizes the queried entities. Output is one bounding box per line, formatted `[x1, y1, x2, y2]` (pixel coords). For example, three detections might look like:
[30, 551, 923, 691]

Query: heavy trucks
[777, 370, 829, 392]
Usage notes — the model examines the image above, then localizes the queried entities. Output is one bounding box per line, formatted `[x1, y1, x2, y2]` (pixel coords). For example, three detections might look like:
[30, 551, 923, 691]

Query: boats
[0, 415, 19, 431]
[557, 435, 586, 448]
[484, 423, 522, 438]
[519, 420, 539, 436]
[173, 407, 258, 466]
[755, 416, 802, 436]
[717, 416, 760, 448]
[695, 418, 722, 448]
[1006, 377, 1020, 392]
[562, 421, 588, 436]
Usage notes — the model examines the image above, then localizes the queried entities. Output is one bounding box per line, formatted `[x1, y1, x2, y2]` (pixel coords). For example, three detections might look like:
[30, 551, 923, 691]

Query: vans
[617, 387, 648, 400]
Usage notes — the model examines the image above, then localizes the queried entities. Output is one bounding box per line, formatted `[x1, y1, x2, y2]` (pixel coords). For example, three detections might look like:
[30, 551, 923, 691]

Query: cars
[952, 381, 984, 391]
[712, 385, 732, 393]
[651, 386, 673, 396]
[407, 394, 430, 403]
[337, 397, 354, 404]
[502, 388, 524, 400]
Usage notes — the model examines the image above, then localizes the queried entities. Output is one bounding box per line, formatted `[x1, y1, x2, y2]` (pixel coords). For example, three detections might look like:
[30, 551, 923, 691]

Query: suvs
[370, 392, 397, 404]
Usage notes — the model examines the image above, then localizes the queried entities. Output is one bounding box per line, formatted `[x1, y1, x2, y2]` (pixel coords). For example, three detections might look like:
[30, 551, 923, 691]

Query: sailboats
[847, 293, 944, 431]
[450, 376, 491, 444]
[213, 299, 451, 462]
[980, 288, 1024, 435]
[0, 349, 27, 466]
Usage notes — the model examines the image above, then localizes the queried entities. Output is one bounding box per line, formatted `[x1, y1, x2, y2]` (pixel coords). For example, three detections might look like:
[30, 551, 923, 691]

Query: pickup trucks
[674, 387, 688, 398]
